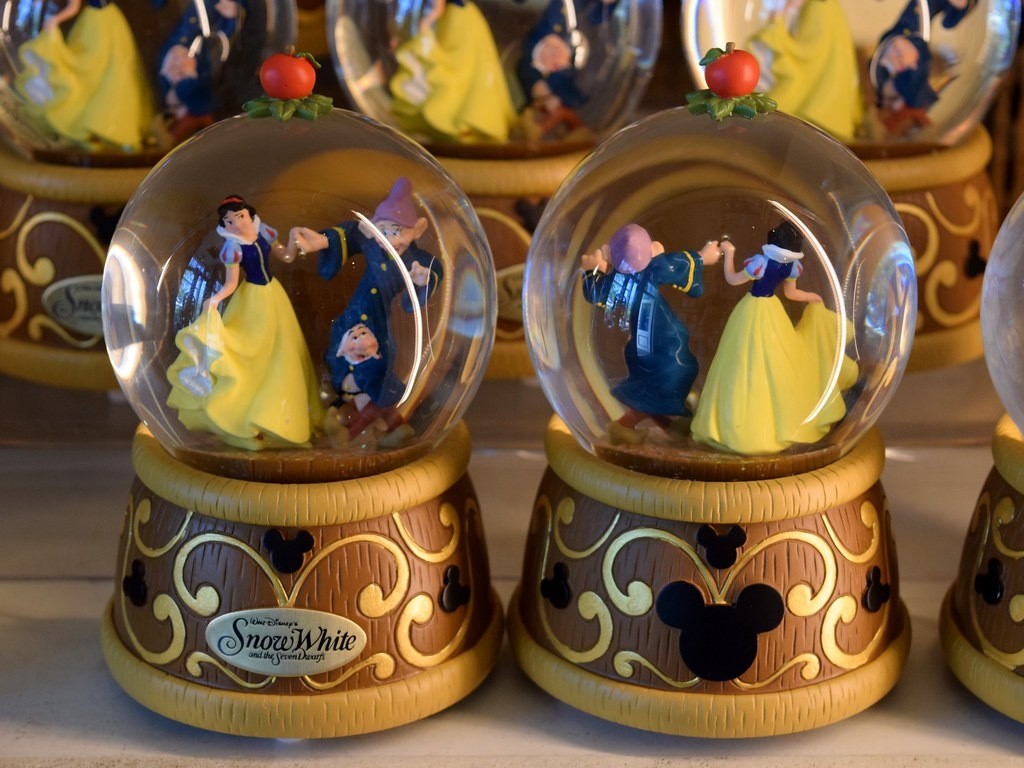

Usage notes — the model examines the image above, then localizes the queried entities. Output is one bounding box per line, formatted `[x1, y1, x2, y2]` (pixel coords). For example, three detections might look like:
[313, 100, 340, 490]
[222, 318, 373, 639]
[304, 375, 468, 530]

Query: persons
[295, 178, 444, 410]
[581, 224, 722, 446]
[167, 194, 325, 450]
[746, 1, 980, 142]
[692, 221, 860, 454]
[388, 1, 621, 146]
[329, 311, 406, 412]
[14, 0, 247, 154]
